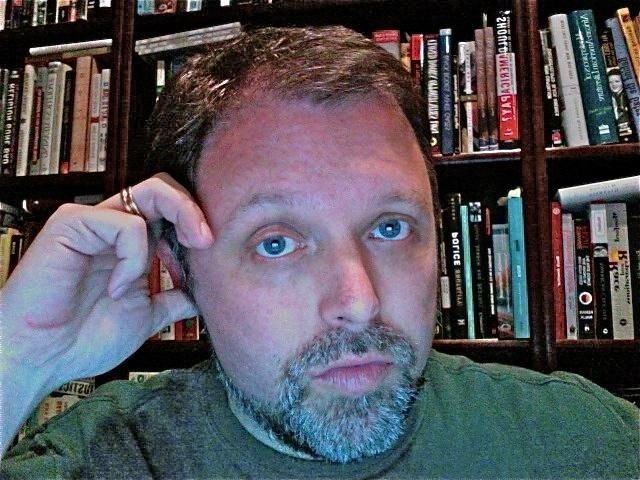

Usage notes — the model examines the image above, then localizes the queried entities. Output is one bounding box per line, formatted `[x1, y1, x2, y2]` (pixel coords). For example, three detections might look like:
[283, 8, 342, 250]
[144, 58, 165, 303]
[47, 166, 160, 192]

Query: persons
[1, 25, 639, 480]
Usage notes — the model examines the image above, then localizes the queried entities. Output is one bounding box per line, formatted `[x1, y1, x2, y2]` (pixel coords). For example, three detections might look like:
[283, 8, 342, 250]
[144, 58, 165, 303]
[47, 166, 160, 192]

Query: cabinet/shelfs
[0, 0, 638, 438]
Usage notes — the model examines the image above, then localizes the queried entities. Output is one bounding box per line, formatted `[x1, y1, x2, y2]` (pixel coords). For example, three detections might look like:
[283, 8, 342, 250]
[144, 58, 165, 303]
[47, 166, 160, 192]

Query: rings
[120, 185, 153, 224]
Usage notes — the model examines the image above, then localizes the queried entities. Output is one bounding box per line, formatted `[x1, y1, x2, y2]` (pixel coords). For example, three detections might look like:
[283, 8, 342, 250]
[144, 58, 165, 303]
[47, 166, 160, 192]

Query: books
[539, 6, 639, 150]
[0, 37, 115, 175]
[2, 196, 108, 292]
[134, 21, 265, 124]
[148, 222, 208, 343]
[4, 0, 115, 33]
[550, 174, 640, 343]
[126, 369, 160, 385]
[18, 377, 97, 445]
[337, 8, 520, 158]
[136, 0, 283, 16]
[433, 185, 531, 342]
